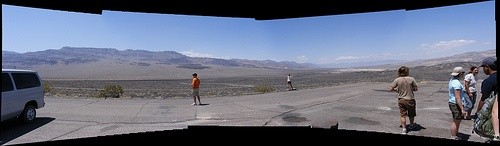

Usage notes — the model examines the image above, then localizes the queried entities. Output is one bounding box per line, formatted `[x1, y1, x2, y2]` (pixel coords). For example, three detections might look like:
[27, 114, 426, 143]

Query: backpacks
[472, 94, 496, 143]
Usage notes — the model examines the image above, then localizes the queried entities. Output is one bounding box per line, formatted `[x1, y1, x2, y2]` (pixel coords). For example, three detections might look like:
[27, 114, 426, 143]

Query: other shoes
[402, 131, 407, 134]
[451, 136, 461, 140]
[191, 103, 196, 105]
[410, 126, 415, 129]
[199, 103, 201, 105]
[465, 116, 472, 120]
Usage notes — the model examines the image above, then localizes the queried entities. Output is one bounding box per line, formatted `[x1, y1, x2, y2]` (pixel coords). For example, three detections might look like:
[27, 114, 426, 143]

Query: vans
[1, 69, 45, 121]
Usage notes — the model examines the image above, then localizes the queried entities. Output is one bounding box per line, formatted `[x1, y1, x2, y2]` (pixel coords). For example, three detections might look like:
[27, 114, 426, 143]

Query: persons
[473, 59, 500, 144]
[448, 67, 468, 140]
[286, 74, 294, 91]
[389, 66, 418, 135]
[464, 66, 478, 120]
[475, 57, 497, 115]
[191, 73, 201, 105]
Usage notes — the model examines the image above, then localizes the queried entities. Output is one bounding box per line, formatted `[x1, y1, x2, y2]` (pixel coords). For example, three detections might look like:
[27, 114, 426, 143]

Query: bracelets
[461, 109, 465, 111]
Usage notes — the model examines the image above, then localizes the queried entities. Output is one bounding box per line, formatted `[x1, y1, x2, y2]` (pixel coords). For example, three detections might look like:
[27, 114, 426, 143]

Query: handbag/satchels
[463, 91, 472, 110]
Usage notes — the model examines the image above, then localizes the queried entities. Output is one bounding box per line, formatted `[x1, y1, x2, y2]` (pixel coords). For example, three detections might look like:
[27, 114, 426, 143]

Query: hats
[451, 67, 465, 76]
[480, 58, 497, 68]
